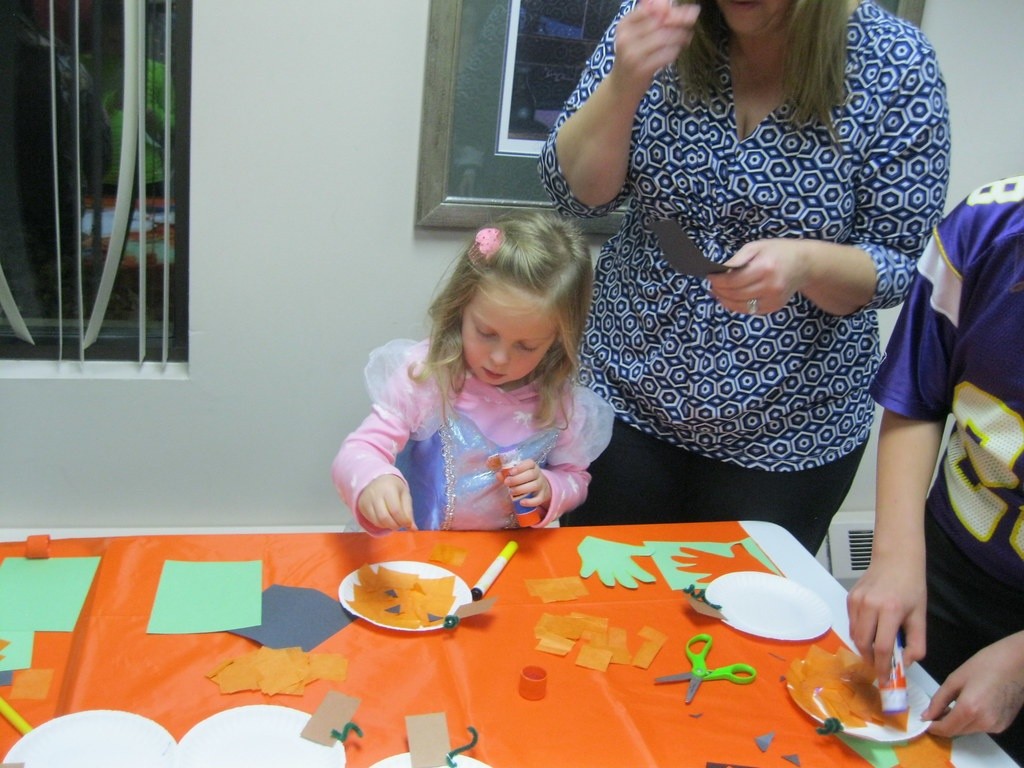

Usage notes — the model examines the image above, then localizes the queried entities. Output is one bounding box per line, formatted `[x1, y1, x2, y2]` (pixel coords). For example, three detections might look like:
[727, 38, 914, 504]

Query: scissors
[655, 633, 757, 705]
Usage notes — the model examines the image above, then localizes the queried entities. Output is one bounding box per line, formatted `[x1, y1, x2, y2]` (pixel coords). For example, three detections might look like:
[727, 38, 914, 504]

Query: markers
[472, 541, 519, 602]
[0, 696, 34, 736]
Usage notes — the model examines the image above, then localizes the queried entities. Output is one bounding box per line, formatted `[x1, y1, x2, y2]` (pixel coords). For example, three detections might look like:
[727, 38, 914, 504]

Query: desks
[0, 520, 1021, 768]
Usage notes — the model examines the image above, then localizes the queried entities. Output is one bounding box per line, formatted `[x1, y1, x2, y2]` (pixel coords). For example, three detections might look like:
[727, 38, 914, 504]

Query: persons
[845, 172, 1024, 768]
[0, 1, 189, 317]
[331, 208, 615, 537]
[537, 0, 952, 556]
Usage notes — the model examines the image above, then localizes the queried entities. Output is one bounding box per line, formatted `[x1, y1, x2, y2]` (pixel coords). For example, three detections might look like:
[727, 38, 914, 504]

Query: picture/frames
[413, 0, 927, 240]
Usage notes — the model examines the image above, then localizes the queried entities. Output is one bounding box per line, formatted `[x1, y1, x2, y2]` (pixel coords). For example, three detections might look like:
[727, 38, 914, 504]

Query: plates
[368, 751, 493, 768]
[338, 560, 473, 631]
[178, 704, 346, 768]
[2, 709, 178, 768]
[705, 571, 832, 641]
[786, 655, 935, 742]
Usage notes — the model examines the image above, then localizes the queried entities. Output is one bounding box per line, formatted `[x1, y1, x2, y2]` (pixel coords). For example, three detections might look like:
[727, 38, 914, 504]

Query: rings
[746, 299, 757, 312]
